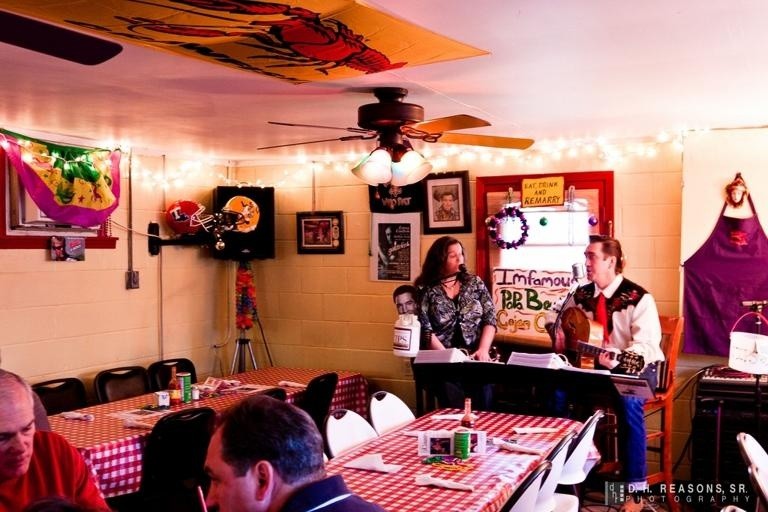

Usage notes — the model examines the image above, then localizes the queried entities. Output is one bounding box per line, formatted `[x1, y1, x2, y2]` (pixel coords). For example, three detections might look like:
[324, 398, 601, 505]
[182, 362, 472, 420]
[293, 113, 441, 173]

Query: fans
[257, 87, 535, 150]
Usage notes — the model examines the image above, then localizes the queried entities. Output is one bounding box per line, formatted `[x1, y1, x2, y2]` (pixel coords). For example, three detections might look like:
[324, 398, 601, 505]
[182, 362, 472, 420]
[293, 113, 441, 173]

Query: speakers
[213, 185, 276, 260]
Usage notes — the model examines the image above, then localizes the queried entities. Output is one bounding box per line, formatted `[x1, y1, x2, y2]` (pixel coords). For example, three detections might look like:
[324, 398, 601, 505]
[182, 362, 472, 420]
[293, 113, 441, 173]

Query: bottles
[461, 398, 475, 429]
[168, 367, 182, 405]
[192, 386, 200, 401]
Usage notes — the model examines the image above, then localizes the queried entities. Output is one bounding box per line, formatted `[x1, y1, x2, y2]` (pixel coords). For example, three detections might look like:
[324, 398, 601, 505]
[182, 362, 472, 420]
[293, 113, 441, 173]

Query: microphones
[552, 283, 579, 352]
[459, 263, 472, 280]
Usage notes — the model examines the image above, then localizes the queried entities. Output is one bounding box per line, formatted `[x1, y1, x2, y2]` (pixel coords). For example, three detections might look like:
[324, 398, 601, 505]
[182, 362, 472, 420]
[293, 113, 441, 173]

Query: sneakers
[619, 496, 643, 512]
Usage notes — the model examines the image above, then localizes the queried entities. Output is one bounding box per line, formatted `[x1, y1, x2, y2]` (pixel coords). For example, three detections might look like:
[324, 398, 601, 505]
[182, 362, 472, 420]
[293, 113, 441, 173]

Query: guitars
[552, 306, 645, 376]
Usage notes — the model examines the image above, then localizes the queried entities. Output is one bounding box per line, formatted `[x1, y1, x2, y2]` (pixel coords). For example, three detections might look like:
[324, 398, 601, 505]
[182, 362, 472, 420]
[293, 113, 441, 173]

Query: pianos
[696, 366, 767, 398]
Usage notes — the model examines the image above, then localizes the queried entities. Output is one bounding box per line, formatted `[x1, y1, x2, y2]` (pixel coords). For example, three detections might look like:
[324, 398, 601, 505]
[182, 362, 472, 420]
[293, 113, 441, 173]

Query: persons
[0, 369, 112, 512]
[551, 235, 665, 512]
[393, 285, 419, 315]
[412, 236, 498, 362]
[433, 192, 460, 221]
[378, 227, 403, 280]
[203, 393, 386, 512]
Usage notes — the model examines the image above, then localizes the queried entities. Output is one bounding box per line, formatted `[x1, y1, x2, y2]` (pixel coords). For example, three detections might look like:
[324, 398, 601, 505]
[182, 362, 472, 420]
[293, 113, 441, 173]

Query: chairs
[33, 316, 768, 512]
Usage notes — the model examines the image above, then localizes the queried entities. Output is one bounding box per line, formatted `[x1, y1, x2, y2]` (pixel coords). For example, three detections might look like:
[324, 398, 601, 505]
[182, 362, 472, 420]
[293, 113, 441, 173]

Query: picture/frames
[422, 170, 472, 235]
[296, 210, 344, 255]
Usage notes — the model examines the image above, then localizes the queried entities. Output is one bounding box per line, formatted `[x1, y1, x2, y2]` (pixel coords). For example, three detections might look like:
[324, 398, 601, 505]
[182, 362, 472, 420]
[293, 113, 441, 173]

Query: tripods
[230, 262, 257, 375]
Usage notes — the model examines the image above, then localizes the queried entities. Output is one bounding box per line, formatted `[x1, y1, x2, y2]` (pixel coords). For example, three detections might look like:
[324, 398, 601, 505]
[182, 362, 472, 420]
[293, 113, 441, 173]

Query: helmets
[222, 196, 260, 233]
[167, 201, 215, 236]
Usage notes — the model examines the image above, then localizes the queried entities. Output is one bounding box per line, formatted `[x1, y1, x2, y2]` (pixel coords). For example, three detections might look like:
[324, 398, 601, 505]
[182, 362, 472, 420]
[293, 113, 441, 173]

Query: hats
[434, 185, 457, 201]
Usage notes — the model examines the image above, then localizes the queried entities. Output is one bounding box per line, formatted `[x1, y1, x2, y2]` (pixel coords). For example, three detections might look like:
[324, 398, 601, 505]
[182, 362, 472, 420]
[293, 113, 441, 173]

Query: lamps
[351, 132, 432, 187]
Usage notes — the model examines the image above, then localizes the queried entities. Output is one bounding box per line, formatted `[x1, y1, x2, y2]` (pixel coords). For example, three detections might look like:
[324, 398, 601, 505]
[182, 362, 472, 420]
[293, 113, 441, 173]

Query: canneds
[454, 429, 470, 459]
[177, 372, 191, 403]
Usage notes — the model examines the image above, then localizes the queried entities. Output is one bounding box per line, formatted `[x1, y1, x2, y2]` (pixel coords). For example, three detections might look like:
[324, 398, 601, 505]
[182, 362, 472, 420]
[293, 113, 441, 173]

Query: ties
[596, 293, 609, 344]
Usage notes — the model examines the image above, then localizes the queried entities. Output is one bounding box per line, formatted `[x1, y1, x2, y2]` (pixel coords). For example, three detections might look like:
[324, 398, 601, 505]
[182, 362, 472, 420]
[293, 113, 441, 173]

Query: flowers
[485, 208, 529, 249]
